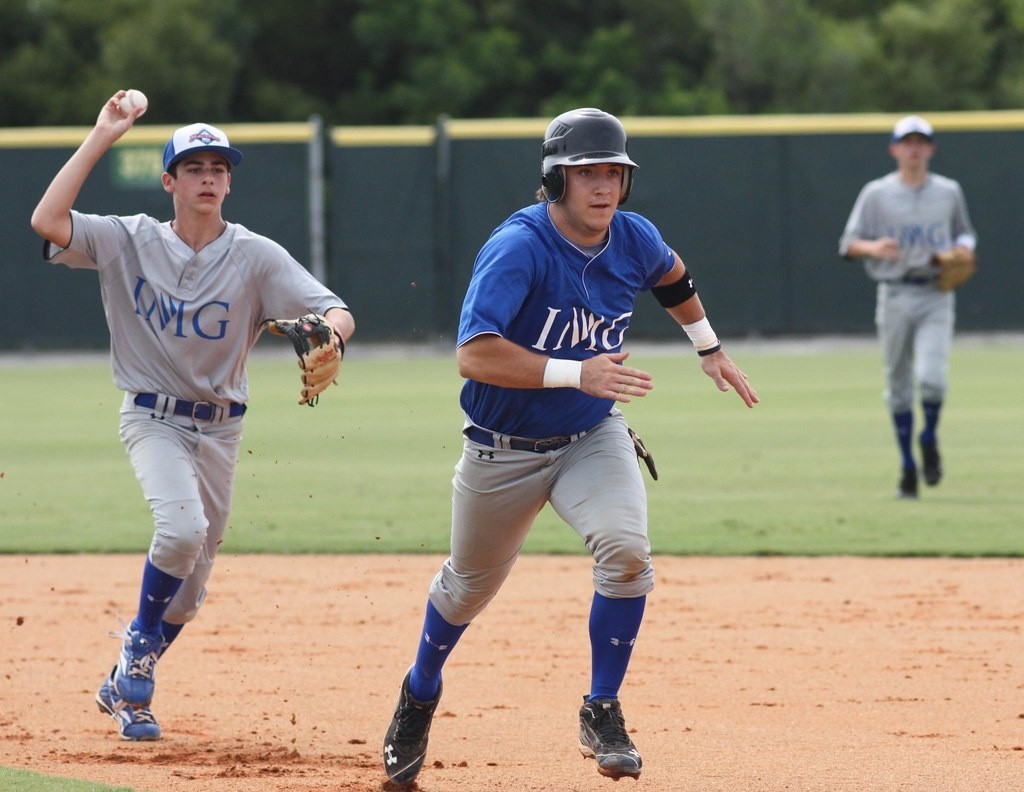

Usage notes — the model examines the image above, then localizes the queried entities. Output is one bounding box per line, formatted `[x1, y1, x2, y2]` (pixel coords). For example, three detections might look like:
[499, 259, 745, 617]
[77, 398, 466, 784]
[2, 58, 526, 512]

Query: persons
[383, 107, 760, 788]
[31, 91, 356, 740]
[838, 116, 976, 498]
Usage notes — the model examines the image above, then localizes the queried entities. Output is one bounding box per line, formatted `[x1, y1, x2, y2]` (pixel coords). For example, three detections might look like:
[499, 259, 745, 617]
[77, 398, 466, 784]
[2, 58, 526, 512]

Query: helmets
[541, 108, 639, 205]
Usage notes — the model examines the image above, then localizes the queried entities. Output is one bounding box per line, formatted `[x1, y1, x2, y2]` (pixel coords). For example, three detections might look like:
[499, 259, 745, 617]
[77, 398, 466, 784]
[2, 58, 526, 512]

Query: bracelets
[680, 316, 719, 351]
[543, 358, 582, 389]
[697, 340, 721, 356]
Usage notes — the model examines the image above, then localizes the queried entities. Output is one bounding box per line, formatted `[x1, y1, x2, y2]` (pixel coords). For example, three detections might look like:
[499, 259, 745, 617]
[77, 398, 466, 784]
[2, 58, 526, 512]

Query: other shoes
[918, 432, 941, 486]
[898, 460, 918, 498]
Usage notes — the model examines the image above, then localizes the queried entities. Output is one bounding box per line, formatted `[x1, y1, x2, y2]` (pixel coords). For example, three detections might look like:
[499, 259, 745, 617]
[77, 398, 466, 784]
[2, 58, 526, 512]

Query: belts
[902, 276, 936, 285]
[134, 392, 246, 420]
[461, 425, 572, 454]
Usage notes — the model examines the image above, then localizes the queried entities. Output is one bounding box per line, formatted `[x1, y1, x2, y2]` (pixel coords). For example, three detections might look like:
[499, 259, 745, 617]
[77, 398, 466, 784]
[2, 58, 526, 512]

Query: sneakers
[107, 612, 168, 706]
[578, 694, 644, 775]
[95, 664, 161, 741]
[383, 664, 443, 786]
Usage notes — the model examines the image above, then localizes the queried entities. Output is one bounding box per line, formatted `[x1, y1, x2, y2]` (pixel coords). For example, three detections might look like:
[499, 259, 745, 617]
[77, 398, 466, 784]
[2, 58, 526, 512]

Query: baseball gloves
[265, 312, 348, 404]
[929, 247, 977, 292]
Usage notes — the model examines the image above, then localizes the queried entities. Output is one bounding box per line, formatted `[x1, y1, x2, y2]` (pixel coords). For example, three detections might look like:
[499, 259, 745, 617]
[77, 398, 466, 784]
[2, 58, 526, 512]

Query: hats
[163, 121, 243, 173]
[890, 115, 936, 144]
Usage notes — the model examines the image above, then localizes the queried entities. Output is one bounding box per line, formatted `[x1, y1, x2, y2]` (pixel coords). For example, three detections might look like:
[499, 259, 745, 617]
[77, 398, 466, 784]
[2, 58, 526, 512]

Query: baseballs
[117, 89, 149, 119]
